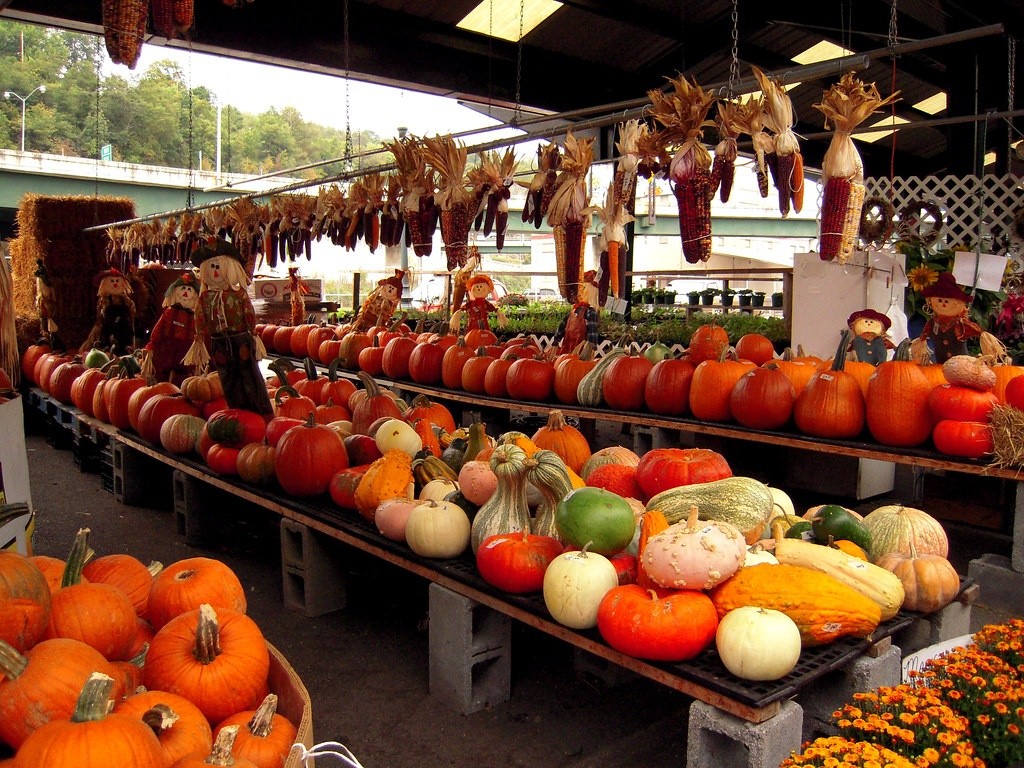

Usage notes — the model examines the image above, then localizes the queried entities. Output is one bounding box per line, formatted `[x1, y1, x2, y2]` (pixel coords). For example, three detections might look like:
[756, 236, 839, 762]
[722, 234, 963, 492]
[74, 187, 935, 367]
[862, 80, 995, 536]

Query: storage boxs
[264, 637, 316, 767]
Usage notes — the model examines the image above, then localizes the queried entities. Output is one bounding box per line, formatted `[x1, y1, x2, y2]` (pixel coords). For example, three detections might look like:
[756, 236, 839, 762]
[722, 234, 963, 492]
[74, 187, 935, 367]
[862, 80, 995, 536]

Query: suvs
[409, 274, 508, 308]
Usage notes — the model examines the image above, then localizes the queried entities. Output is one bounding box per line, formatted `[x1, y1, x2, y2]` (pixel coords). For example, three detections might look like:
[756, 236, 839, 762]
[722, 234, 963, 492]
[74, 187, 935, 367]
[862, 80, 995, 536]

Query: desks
[24, 355, 1024, 767]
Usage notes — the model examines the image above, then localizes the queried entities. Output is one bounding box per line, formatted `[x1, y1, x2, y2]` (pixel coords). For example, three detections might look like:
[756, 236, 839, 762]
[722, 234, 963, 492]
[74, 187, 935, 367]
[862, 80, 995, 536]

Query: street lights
[5, 85, 47, 151]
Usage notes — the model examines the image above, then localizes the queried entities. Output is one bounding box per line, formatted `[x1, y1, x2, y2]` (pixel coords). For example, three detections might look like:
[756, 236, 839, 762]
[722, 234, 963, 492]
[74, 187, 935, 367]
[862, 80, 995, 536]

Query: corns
[107, 185, 509, 325]
[522, 149, 866, 305]
[102, 0, 194, 70]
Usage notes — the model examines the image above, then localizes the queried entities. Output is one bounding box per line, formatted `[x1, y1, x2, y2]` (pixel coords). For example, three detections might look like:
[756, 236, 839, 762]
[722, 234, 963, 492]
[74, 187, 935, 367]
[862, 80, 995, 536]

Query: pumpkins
[23, 340, 959, 681]
[0, 528, 300, 768]
[254, 310, 1024, 457]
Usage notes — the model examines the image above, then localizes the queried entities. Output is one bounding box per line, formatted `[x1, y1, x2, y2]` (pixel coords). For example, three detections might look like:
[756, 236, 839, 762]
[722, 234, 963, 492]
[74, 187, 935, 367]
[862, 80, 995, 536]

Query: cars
[663, 277, 723, 307]
[519, 287, 562, 304]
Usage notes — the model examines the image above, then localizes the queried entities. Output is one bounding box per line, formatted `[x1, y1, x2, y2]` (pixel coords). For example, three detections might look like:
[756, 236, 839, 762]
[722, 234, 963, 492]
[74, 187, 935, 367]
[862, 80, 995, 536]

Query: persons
[181, 240, 275, 447]
[353, 268, 406, 332]
[453, 252, 482, 315]
[34, 259, 68, 357]
[140, 271, 211, 389]
[911, 273, 1007, 366]
[284, 267, 310, 326]
[448, 275, 509, 335]
[549, 270, 602, 362]
[846, 309, 910, 367]
[79, 263, 150, 357]
